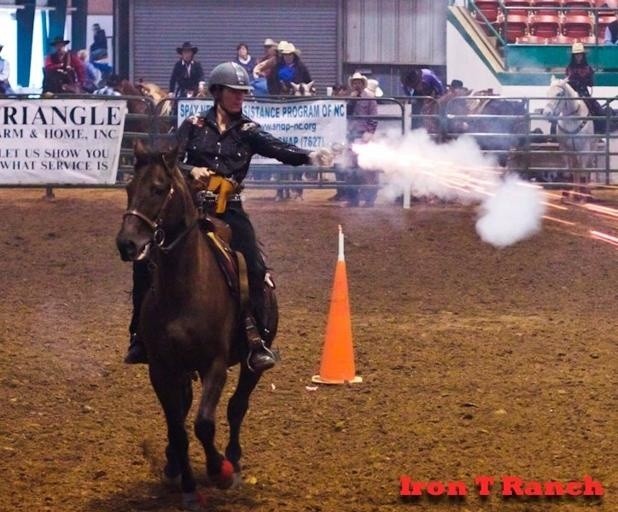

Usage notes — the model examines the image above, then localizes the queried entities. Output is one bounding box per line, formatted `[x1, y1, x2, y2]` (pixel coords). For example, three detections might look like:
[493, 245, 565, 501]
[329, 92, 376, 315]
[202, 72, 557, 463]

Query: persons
[124, 60, 334, 370]
[604, 11, 618, 45]
[547, 43, 604, 144]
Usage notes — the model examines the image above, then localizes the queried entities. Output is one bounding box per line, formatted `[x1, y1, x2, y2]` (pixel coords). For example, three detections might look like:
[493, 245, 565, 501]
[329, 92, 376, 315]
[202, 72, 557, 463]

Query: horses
[272, 80, 317, 202]
[48, 52, 82, 93]
[114, 137, 278, 511]
[421, 91, 532, 161]
[110, 77, 169, 134]
[542, 73, 598, 204]
[135, 81, 175, 117]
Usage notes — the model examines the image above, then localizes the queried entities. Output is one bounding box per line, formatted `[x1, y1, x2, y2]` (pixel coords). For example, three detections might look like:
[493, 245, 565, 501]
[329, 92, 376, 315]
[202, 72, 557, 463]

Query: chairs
[464, 0, 618, 73]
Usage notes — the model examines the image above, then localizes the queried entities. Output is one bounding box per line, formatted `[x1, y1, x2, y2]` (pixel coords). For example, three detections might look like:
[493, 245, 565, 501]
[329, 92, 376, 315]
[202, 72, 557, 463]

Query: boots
[245, 292, 276, 370]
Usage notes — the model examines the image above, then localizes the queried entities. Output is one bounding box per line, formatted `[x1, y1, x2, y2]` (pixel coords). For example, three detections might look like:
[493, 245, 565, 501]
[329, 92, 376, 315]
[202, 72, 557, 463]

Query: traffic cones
[311, 223, 366, 386]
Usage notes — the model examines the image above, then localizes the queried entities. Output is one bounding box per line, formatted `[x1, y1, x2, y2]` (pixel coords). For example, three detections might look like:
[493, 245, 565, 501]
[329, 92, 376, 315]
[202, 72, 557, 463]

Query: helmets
[207, 61, 256, 93]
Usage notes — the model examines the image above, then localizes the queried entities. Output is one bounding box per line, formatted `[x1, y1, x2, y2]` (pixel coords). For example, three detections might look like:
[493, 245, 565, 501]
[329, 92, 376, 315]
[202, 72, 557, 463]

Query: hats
[263, 38, 302, 56]
[570, 41, 587, 57]
[49, 36, 70, 45]
[348, 72, 369, 89]
[175, 40, 199, 54]
